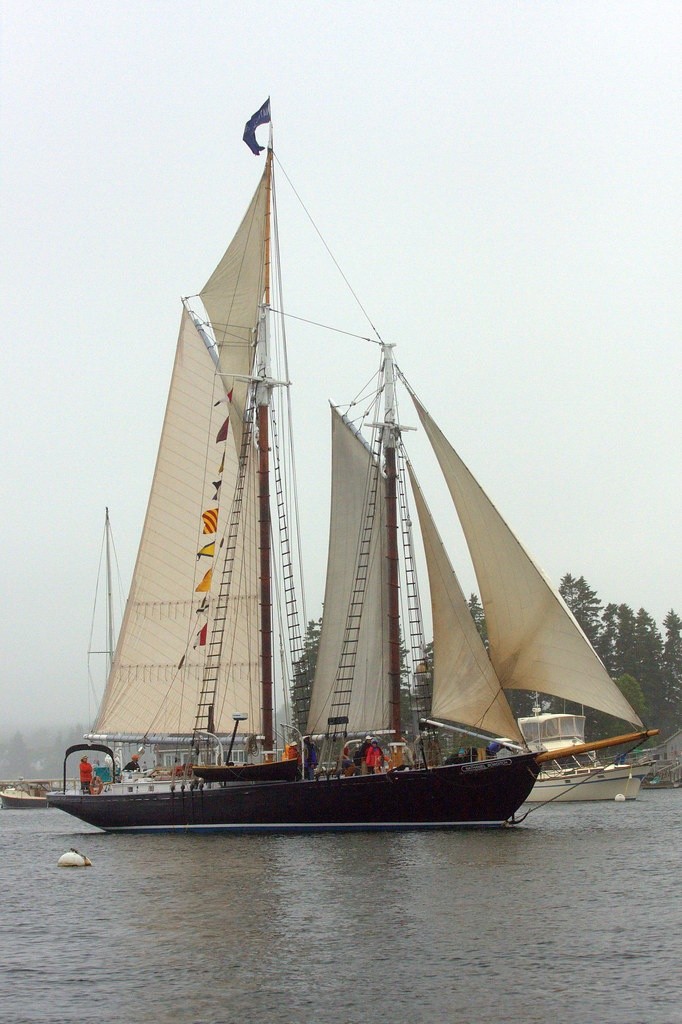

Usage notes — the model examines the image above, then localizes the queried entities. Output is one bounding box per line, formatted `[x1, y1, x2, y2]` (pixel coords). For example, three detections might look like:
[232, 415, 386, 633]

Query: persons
[80, 755, 93, 790]
[342, 735, 385, 776]
[122, 754, 148, 779]
[289, 737, 316, 781]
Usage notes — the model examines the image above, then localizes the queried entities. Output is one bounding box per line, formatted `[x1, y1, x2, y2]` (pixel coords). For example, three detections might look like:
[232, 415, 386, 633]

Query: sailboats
[46, 95, 660, 837]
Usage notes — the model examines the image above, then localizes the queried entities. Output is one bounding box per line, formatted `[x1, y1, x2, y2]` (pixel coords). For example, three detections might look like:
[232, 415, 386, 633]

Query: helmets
[290, 741, 297, 746]
[132, 754, 139, 758]
[302, 736, 309, 740]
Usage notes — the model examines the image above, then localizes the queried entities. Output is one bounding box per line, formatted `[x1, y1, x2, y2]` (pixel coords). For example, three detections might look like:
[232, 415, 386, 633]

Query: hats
[81, 755, 88, 759]
[366, 736, 373, 739]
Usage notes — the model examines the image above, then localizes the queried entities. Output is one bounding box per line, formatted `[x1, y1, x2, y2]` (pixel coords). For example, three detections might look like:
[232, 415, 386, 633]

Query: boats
[0, 788, 47, 808]
[488, 690, 657, 802]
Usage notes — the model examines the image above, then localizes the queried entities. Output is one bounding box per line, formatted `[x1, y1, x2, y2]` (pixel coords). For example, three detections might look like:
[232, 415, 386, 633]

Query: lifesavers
[89, 777, 103, 795]
[373, 755, 393, 774]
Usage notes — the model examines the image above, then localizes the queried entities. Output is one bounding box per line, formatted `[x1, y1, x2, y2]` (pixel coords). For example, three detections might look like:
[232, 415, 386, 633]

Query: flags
[193, 387, 234, 648]
[243, 97, 271, 156]
